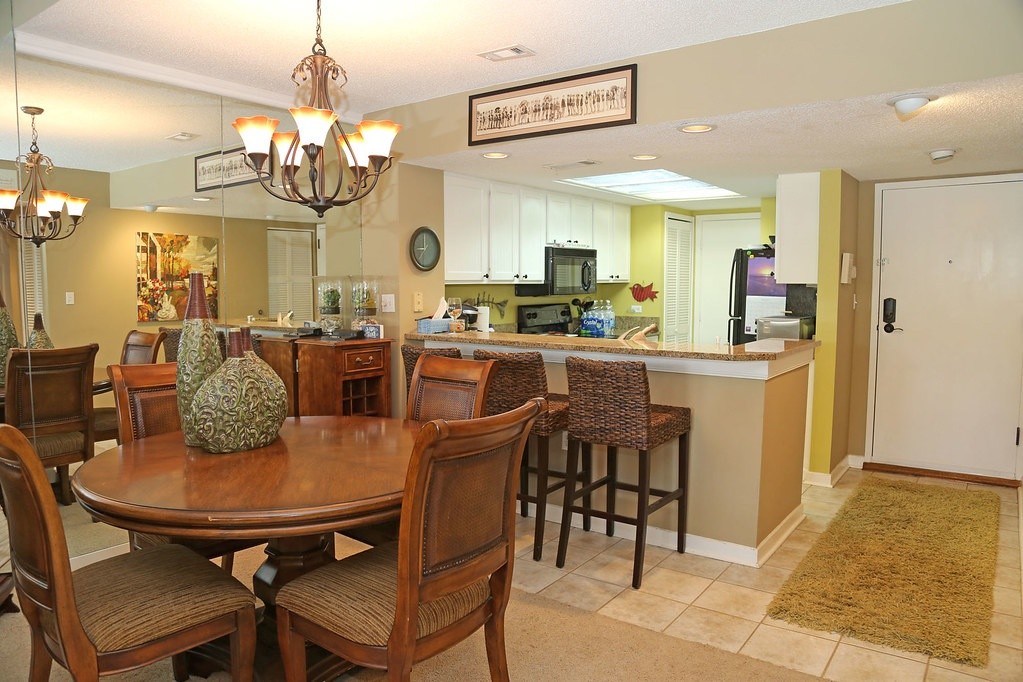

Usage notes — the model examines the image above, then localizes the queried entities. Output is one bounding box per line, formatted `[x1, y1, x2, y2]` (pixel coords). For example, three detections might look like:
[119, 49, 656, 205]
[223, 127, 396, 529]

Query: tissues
[416, 296, 454, 334]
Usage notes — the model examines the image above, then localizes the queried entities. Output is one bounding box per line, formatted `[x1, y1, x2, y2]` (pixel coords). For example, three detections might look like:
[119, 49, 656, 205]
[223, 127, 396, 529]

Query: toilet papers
[475, 306, 490, 333]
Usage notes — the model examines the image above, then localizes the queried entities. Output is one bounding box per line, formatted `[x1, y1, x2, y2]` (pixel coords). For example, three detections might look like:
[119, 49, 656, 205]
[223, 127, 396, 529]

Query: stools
[398, 344, 692, 589]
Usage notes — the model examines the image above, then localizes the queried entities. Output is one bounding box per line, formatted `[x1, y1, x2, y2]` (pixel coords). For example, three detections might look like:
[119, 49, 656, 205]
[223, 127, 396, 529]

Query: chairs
[6, 330, 168, 503]
[0, 353, 549, 682]
[159, 327, 263, 363]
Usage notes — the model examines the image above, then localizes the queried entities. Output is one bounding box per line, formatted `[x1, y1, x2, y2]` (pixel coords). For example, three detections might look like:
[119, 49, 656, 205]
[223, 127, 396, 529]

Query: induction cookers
[517, 303, 619, 339]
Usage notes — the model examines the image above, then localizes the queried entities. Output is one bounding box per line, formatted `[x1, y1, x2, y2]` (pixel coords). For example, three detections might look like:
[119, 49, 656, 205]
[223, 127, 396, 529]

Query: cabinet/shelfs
[775, 172, 820, 285]
[295, 336, 395, 417]
[257, 336, 298, 418]
[445, 171, 632, 284]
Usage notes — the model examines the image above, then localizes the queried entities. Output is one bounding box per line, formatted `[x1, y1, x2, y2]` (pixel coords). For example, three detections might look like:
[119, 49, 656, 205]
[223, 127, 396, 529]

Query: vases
[178, 269, 222, 448]
[190, 327, 285, 451]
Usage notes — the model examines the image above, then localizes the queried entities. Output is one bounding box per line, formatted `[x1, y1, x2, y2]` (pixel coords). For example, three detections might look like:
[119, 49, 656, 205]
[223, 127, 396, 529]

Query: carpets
[765, 476, 1000, 670]
[1, 449, 835, 682]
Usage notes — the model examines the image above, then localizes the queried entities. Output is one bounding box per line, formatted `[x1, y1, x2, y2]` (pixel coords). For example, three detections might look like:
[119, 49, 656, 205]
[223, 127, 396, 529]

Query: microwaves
[755, 315, 814, 341]
[514, 250, 597, 296]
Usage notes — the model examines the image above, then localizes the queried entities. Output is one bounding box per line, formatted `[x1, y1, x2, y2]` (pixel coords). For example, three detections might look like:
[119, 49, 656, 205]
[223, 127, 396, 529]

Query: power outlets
[66, 292, 75, 305]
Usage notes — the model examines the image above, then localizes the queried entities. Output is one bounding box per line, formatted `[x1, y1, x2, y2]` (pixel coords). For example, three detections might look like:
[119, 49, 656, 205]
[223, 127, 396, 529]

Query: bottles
[0, 289, 19, 388]
[175, 272, 289, 454]
[25, 313, 54, 350]
[580, 300, 616, 335]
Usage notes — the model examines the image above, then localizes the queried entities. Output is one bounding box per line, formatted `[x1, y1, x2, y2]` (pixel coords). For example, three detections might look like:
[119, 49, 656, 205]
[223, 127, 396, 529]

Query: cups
[448, 321, 460, 331]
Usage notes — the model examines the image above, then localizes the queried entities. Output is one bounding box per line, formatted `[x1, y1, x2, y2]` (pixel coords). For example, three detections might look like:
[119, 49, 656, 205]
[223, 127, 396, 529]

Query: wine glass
[447, 298, 462, 335]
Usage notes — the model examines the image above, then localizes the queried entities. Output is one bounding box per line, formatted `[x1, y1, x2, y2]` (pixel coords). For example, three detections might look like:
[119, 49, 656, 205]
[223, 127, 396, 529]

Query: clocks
[410, 226, 441, 271]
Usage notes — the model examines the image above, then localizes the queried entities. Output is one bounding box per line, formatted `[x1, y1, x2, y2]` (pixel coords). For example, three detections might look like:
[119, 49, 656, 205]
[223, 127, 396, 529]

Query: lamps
[0, 106, 90, 248]
[230, 0, 403, 218]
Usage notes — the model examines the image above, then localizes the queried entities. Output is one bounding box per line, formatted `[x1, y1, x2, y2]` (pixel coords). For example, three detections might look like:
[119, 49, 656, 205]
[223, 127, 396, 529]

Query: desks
[69, 417, 425, 682]
[0, 367, 112, 504]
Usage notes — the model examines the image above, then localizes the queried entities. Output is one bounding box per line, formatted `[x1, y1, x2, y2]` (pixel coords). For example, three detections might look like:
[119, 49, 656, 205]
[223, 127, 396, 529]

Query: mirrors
[0, 2, 362, 460]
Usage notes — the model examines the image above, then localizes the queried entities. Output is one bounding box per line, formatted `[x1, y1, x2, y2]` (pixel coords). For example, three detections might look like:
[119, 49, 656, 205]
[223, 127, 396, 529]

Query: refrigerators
[727, 249, 816, 346]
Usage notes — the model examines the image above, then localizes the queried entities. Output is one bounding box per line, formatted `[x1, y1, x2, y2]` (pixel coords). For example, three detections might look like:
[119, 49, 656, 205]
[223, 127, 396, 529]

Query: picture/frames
[468, 64, 637, 146]
